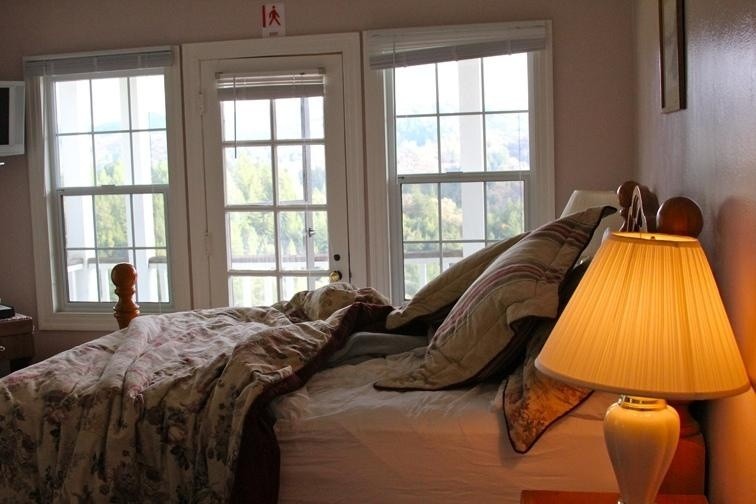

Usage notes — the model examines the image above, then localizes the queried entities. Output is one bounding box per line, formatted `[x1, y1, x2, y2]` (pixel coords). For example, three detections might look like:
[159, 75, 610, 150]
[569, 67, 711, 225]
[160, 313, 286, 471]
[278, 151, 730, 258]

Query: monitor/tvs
[0, 80, 26, 157]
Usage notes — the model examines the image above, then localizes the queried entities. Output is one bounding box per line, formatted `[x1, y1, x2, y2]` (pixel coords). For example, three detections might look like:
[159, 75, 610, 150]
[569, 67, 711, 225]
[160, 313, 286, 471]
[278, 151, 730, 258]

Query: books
[0, 303, 15, 318]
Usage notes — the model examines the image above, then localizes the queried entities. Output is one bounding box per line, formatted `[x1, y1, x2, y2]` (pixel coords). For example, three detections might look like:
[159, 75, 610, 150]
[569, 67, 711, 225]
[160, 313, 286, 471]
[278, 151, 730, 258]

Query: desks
[0, 312, 36, 376]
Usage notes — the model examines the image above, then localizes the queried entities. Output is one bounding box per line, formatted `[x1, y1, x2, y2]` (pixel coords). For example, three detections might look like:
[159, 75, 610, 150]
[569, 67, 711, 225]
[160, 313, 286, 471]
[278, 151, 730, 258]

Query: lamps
[561, 188, 623, 260]
[533, 226, 751, 504]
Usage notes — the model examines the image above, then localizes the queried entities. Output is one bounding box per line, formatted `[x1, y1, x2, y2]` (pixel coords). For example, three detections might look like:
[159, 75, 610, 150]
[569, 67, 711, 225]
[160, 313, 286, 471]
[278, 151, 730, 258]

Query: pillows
[383, 232, 528, 332]
[373, 204, 617, 391]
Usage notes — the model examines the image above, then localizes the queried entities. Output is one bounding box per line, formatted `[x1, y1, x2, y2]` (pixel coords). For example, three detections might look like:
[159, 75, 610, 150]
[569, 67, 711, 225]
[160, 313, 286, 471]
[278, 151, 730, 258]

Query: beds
[0, 179, 756, 504]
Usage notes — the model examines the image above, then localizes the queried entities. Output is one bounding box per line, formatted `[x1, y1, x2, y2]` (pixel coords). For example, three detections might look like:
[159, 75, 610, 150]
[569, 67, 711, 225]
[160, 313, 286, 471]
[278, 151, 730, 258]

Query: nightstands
[519, 489, 714, 504]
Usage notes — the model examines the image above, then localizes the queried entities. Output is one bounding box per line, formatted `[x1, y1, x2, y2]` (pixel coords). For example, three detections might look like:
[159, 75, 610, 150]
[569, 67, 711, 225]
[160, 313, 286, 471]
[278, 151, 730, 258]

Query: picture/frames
[657, 0, 687, 115]
